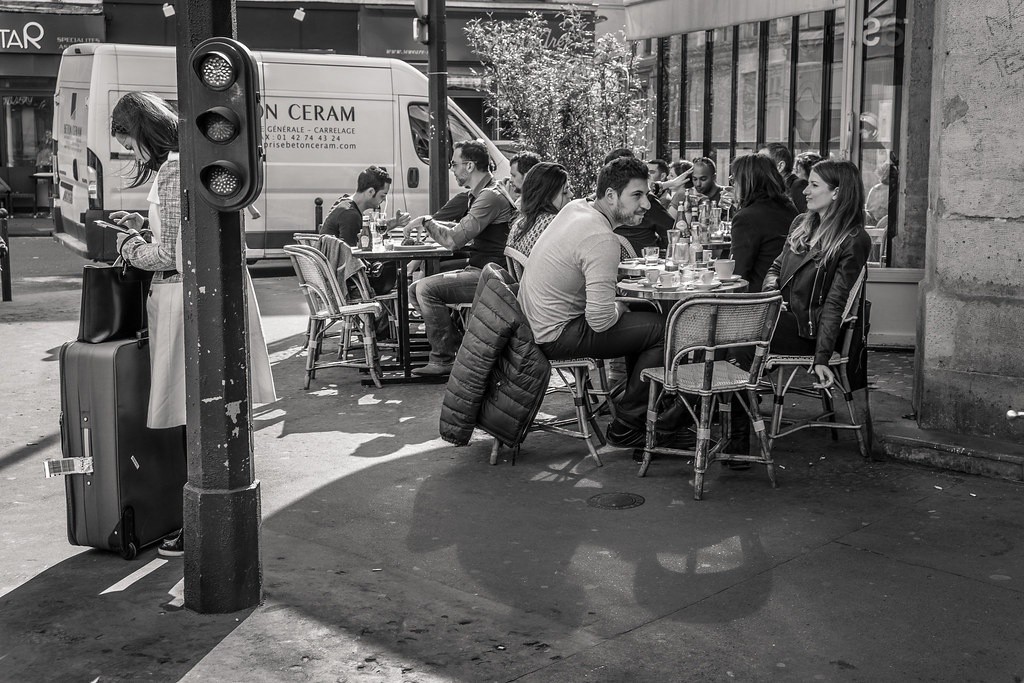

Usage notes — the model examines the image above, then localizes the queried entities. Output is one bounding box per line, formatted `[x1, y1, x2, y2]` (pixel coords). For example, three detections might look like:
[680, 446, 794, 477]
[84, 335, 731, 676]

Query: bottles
[672, 188, 732, 263]
[359, 215, 373, 251]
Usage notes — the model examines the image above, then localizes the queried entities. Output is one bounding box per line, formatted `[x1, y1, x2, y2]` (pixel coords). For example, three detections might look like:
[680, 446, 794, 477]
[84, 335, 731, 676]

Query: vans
[48, 42, 512, 268]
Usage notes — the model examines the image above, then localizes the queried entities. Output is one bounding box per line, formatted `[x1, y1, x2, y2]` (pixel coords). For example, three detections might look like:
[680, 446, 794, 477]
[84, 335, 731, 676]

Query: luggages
[59, 330, 182, 560]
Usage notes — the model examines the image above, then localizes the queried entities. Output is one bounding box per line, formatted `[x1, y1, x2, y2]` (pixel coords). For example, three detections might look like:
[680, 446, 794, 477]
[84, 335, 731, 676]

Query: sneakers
[157, 528, 184, 556]
[605, 416, 676, 448]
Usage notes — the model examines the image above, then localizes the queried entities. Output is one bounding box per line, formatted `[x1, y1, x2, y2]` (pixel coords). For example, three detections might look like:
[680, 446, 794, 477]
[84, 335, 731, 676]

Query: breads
[401, 237, 424, 245]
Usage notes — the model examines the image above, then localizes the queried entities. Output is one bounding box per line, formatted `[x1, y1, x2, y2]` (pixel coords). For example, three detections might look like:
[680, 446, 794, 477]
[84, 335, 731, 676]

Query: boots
[655, 392, 700, 433]
[721, 412, 752, 464]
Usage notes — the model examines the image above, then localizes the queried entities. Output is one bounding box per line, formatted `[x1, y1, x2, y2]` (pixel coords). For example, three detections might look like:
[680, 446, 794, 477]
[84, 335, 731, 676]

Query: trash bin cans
[29, 174, 54, 208]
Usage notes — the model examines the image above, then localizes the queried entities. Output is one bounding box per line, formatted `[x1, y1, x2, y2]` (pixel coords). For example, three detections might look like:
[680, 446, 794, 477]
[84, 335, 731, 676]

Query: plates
[692, 281, 721, 289]
[393, 245, 436, 250]
[713, 274, 742, 282]
[637, 279, 658, 287]
[652, 283, 683, 290]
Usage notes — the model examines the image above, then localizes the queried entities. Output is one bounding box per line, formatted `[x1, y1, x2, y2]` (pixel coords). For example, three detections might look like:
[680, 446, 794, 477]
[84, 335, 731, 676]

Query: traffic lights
[186, 36, 264, 212]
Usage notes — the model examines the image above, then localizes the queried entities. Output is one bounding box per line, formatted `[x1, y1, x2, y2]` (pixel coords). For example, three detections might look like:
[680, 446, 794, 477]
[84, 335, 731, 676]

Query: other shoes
[411, 364, 453, 375]
[379, 343, 393, 350]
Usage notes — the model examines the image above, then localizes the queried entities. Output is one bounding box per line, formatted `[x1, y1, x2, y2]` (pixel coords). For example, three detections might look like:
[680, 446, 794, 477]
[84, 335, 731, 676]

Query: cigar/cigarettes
[824, 388, 832, 399]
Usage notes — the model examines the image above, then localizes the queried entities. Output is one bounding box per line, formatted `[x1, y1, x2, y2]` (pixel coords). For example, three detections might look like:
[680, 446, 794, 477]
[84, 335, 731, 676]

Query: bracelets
[422, 215, 432, 226]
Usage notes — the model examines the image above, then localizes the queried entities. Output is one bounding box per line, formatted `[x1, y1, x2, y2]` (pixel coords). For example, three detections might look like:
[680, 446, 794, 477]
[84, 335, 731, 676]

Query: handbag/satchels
[77, 255, 154, 344]
[835, 264, 872, 392]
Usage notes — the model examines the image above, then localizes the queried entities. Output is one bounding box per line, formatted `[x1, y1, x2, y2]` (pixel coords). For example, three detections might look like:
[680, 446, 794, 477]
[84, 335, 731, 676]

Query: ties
[468, 194, 473, 208]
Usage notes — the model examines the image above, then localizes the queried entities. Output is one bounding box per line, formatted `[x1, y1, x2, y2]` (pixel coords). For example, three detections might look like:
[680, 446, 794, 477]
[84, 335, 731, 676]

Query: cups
[718, 186, 734, 210]
[637, 229, 735, 291]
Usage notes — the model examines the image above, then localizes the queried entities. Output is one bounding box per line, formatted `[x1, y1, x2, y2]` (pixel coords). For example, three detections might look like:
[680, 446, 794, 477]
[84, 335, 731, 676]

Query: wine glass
[375, 213, 387, 247]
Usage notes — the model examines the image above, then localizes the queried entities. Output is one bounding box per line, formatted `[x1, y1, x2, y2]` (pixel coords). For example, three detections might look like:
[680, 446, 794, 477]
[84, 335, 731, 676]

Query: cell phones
[94, 220, 128, 233]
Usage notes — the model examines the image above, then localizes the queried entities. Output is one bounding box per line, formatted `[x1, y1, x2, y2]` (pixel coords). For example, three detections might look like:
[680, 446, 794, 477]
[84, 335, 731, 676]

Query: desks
[386, 231, 428, 238]
[617, 278, 751, 448]
[681, 236, 733, 257]
[351, 246, 455, 384]
[357, 225, 426, 233]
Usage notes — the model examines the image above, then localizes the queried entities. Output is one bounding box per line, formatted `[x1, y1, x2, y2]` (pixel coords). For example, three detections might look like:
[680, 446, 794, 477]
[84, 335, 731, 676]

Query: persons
[105, 92, 277, 558]
[320, 167, 412, 347]
[40, 129, 52, 171]
[403, 138, 872, 464]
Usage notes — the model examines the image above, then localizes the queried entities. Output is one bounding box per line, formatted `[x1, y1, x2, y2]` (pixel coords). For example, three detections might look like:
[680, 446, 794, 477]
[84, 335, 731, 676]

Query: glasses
[729, 174, 735, 186]
[693, 158, 711, 164]
[451, 160, 478, 167]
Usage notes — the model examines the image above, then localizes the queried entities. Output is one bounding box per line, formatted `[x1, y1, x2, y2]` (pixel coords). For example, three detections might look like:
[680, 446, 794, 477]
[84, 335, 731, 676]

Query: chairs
[283, 232, 401, 389]
[483, 262, 605, 468]
[634, 289, 785, 502]
[763, 262, 868, 458]
